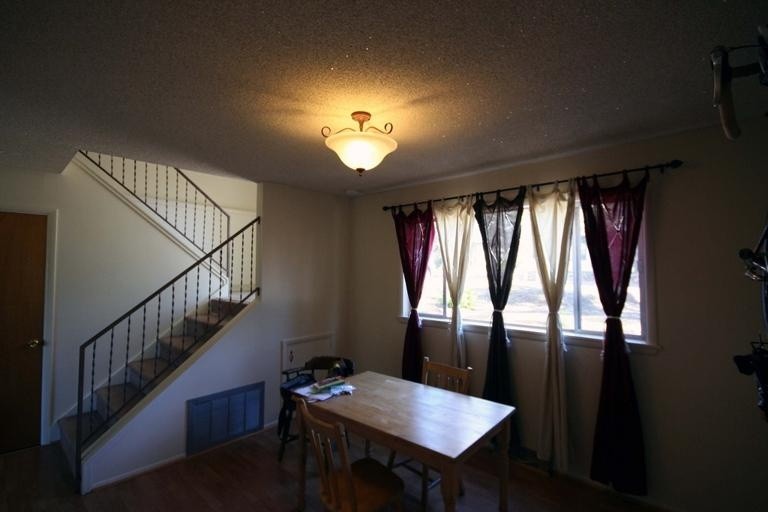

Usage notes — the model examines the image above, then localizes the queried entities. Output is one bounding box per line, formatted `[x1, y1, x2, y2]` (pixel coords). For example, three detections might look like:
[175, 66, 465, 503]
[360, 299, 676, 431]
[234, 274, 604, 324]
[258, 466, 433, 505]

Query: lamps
[323, 110, 398, 179]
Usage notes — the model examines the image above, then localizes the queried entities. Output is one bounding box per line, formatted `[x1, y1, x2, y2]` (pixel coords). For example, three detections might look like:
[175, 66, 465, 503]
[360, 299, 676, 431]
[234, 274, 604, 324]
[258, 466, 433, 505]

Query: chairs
[294, 350, 473, 510]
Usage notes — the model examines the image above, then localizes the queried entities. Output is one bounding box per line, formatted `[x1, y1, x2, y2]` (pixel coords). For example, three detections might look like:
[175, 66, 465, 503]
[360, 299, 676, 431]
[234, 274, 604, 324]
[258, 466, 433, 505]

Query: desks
[289, 368, 519, 510]
